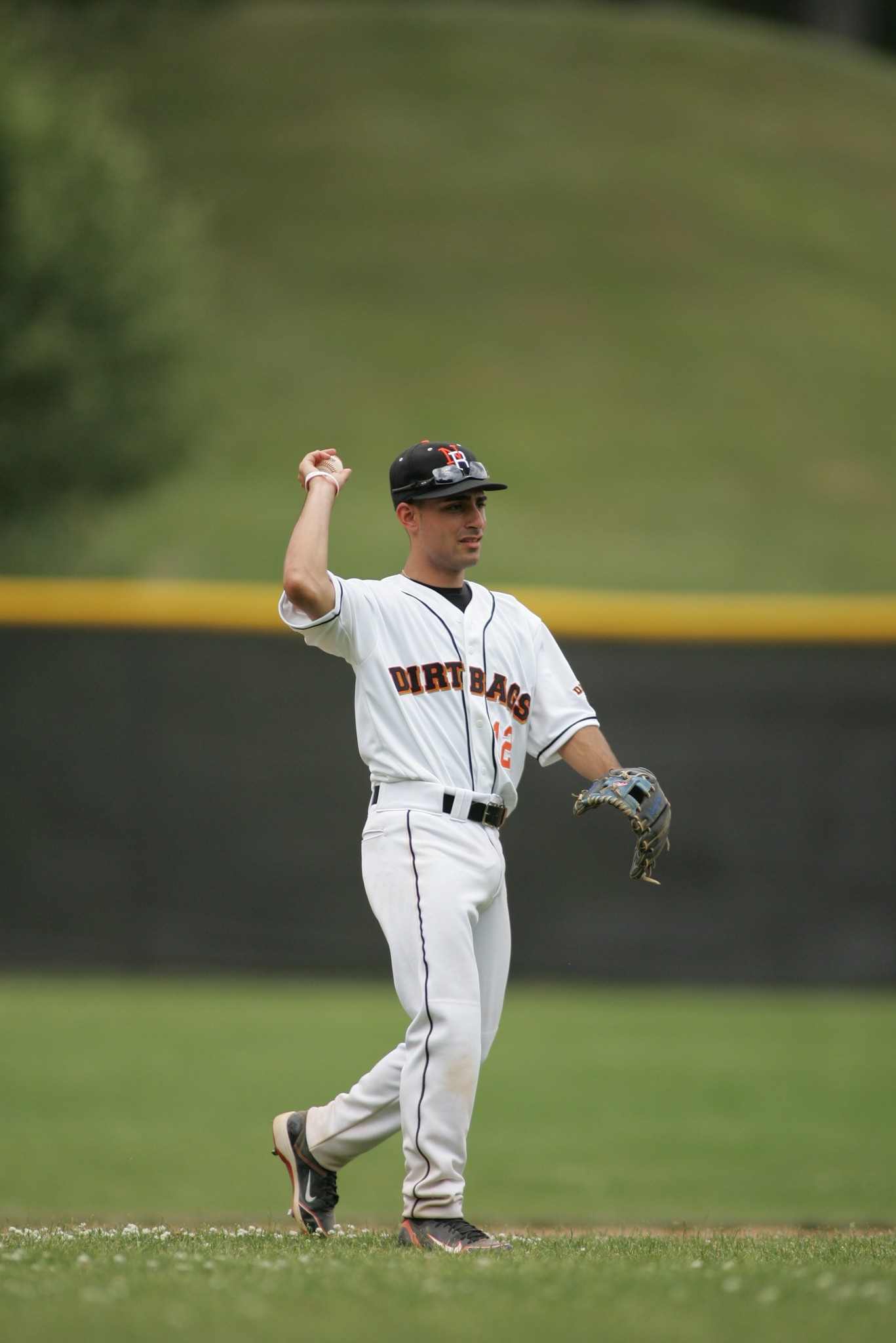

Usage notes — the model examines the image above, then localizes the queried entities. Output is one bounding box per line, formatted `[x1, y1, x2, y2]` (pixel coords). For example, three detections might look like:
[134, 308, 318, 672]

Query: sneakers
[274, 1108, 340, 1240]
[395, 1213, 514, 1255]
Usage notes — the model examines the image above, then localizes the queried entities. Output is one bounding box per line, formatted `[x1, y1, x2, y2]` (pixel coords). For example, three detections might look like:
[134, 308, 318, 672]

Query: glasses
[390, 461, 491, 494]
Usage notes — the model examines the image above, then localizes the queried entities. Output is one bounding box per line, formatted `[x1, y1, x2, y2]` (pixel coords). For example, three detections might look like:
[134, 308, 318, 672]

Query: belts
[370, 785, 507, 830]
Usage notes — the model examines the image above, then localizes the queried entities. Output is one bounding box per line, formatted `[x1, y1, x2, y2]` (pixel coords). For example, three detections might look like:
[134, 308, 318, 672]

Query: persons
[272, 438, 626, 1257]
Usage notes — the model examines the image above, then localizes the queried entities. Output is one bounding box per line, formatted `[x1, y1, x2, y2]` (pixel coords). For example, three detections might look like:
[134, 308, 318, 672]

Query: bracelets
[304, 471, 342, 498]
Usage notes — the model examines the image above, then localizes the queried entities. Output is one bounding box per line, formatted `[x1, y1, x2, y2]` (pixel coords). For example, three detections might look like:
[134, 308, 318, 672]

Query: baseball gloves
[574, 766, 673, 883]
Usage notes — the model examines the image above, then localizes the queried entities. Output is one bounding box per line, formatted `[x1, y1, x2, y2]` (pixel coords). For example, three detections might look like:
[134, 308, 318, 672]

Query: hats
[390, 439, 508, 507]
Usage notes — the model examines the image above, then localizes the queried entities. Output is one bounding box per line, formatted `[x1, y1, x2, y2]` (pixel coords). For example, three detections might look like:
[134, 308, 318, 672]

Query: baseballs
[316, 455, 342, 476]
